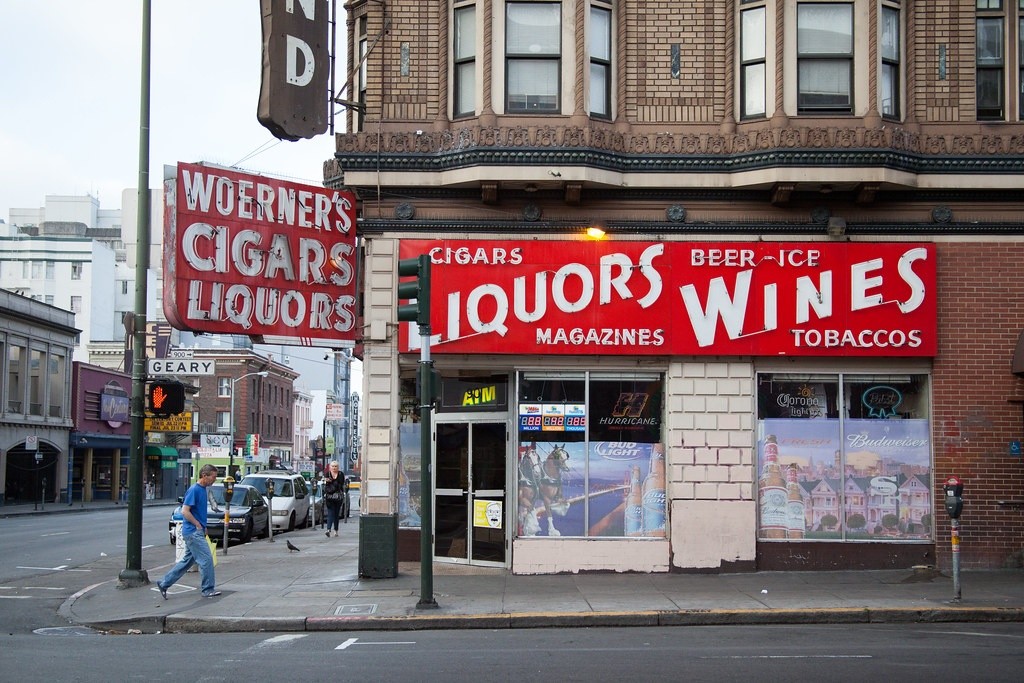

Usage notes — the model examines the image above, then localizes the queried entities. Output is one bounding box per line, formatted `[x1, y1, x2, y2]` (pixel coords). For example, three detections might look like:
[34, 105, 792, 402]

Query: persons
[155, 464, 223, 600]
[326, 460, 345, 538]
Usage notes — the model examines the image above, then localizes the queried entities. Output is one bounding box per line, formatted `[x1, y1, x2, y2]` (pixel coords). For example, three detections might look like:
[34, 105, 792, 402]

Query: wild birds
[286, 539, 300, 553]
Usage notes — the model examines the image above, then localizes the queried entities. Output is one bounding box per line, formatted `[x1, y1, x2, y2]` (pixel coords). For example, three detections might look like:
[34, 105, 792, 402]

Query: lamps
[827, 216, 846, 237]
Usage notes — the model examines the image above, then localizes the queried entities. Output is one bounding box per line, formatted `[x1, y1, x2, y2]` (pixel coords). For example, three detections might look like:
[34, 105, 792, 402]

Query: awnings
[147, 445, 179, 469]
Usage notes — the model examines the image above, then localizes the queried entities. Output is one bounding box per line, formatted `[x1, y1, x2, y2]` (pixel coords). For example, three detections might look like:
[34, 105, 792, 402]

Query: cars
[304, 480, 350, 523]
[238, 470, 309, 532]
[169, 483, 268, 545]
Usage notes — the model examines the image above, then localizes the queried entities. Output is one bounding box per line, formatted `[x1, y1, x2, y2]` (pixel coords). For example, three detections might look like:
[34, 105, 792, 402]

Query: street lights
[343, 477, 351, 523]
[229, 371, 269, 476]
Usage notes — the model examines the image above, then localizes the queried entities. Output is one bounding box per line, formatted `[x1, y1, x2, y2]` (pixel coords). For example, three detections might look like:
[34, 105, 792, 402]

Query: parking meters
[222, 475, 237, 555]
[310, 477, 319, 529]
[264, 477, 276, 541]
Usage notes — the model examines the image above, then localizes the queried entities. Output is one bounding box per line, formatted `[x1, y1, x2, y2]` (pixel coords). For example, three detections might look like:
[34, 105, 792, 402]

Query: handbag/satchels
[205, 534, 217, 567]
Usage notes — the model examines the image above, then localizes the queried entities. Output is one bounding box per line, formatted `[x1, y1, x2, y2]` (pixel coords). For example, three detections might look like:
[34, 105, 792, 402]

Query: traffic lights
[395, 255, 431, 330]
[229, 441, 239, 456]
[148, 379, 184, 415]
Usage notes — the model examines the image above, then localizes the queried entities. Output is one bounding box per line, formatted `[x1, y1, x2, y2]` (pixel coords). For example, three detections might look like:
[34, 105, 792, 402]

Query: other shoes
[157, 581, 169, 600]
[325, 531, 330, 537]
[334, 531, 338, 537]
[202, 591, 221, 596]
